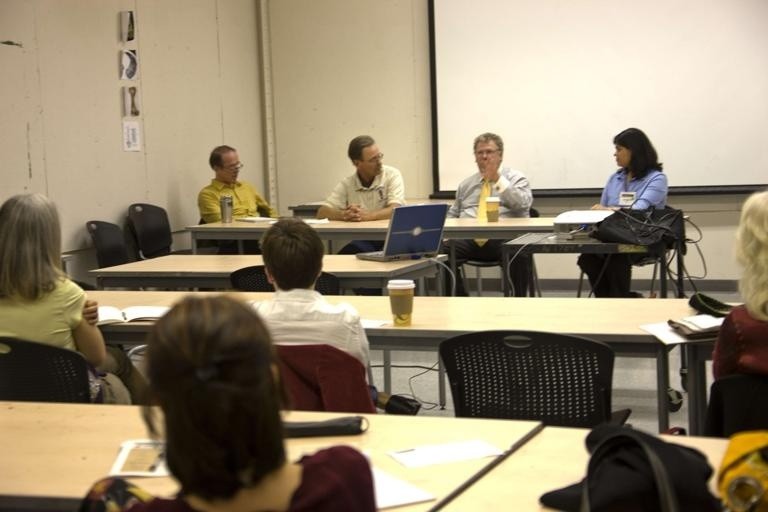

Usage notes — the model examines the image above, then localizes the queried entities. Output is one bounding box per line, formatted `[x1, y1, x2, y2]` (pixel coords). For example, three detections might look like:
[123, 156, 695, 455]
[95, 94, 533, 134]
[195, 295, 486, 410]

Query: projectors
[552, 209, 617, 235]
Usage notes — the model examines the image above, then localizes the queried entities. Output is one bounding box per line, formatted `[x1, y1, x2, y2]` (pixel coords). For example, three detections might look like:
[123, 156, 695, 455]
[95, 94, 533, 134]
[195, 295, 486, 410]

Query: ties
[474, 178, 491, 248]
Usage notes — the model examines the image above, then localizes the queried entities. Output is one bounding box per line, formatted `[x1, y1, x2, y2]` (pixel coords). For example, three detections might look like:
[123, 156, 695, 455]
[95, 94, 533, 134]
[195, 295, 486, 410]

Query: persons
[249, 216, 421, 416]
[196, 146, 278, 254]
[0, 191, 146, 405]
[316, 134, 405, 294]
[576, 128, 668, 297]
[712, 188, 767, 379]
[77, 296, 378, 512]
[434, 131, 534, 297]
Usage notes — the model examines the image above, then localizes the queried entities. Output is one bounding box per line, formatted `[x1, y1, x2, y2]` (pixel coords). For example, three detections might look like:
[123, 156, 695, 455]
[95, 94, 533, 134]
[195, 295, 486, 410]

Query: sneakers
[385, 395, 421, 415]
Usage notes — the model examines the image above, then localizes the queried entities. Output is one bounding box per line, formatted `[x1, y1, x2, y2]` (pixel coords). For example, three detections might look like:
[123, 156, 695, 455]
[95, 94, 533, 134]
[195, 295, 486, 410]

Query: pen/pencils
[122, 312, 127, 320]
[149, 452, 164, 473]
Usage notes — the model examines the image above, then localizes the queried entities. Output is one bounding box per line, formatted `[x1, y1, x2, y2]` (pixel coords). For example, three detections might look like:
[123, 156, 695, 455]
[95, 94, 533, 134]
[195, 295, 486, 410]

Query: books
[553, 209, 616, 225]
[96, 303, 171, 326]
[235, 216, 273, 223]
[373, 465, 437, 510]
[639, 314, 726, 346]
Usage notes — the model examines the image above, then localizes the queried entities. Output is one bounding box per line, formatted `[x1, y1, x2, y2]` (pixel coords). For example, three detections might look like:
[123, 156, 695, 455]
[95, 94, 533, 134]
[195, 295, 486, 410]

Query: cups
[486, 196, 501, 221]
[219, 194, 233, 223]
[387, 279, 416, 327]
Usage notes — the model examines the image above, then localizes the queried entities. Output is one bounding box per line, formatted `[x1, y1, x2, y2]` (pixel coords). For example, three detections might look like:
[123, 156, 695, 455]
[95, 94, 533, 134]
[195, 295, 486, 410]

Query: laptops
[355, 202, 453, 262]
